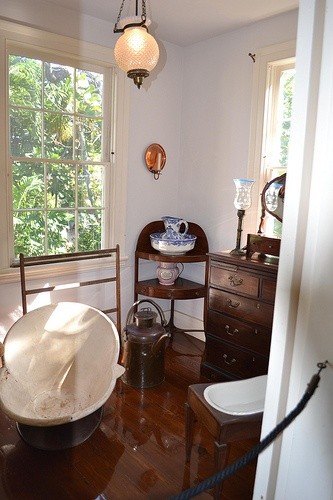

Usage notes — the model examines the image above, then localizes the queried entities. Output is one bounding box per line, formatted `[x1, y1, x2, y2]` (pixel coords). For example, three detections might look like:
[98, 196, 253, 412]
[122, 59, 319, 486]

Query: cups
[161, 216, 189, 235]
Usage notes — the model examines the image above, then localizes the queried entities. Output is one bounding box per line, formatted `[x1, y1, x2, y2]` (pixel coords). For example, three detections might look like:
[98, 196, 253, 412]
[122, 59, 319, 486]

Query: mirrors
[261, 173, 287, 223]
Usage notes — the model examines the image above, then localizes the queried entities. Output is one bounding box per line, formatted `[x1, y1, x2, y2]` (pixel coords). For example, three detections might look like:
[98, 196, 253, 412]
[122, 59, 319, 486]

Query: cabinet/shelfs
[132, 218, 213, 359]
[200, 249, 279, 384]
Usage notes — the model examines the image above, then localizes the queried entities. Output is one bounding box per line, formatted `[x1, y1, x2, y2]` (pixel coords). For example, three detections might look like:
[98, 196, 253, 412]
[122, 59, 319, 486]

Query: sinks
[203, 373, 268, 416]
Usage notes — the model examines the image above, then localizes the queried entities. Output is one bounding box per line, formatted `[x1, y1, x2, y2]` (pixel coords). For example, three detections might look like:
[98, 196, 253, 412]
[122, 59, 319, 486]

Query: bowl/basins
[150, 233, 197, 255]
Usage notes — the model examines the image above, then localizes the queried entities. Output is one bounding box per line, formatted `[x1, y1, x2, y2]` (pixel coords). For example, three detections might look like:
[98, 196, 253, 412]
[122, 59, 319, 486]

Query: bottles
[156, 262, 184, 285]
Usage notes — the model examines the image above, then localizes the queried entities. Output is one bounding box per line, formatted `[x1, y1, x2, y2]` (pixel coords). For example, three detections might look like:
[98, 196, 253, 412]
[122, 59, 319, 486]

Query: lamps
[112, 0, 162, 90]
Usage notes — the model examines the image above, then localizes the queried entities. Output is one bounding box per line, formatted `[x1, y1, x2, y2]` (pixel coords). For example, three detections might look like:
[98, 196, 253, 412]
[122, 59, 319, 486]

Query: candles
[156, 152, 162, 171]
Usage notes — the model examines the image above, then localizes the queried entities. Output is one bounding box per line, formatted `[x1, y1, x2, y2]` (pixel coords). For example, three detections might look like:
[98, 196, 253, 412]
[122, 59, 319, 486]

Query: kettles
[123, 298, 171, 388]
